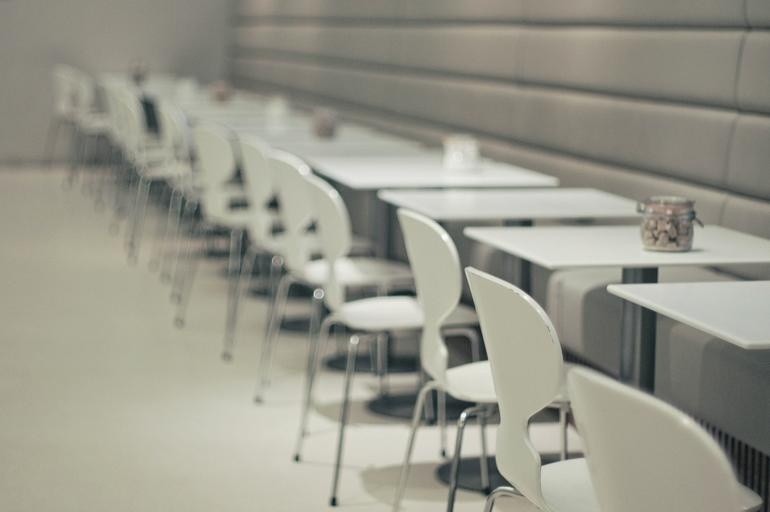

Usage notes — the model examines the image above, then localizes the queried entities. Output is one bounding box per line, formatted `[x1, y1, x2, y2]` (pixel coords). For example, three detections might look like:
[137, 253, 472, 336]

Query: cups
[443, 137, 481, 174]
[126, 63, 290, 124]
[313, 107, 338, 139]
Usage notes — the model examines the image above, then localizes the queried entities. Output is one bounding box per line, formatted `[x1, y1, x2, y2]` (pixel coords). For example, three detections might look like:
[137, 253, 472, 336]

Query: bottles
[637, 193, 704, 252]
